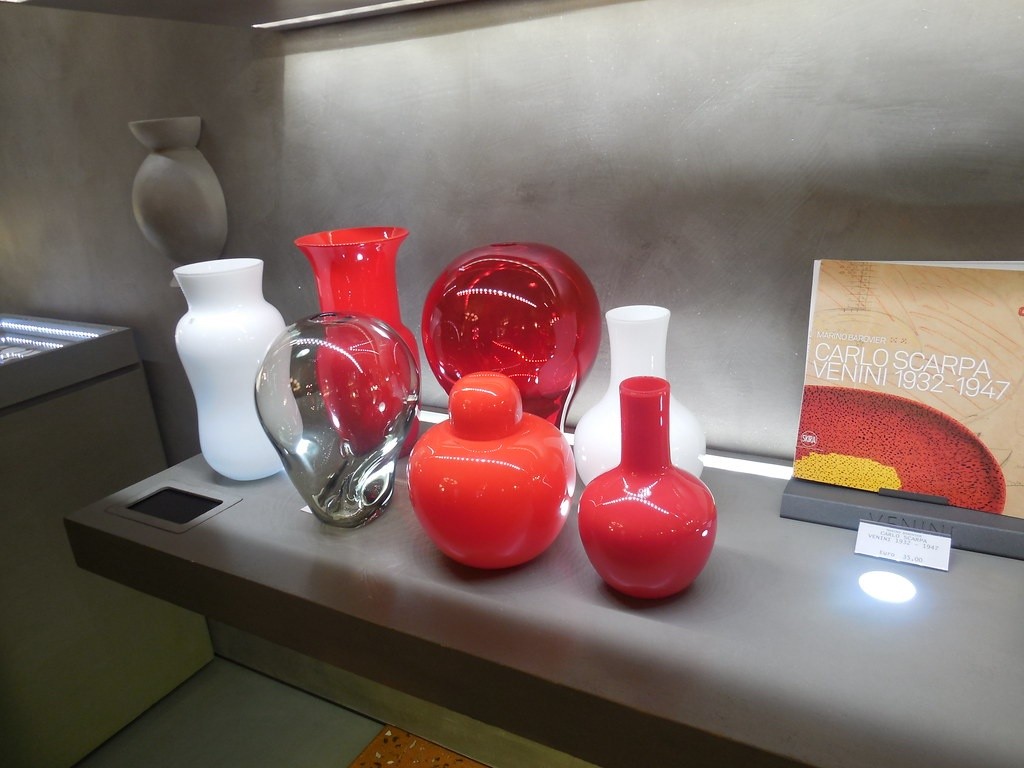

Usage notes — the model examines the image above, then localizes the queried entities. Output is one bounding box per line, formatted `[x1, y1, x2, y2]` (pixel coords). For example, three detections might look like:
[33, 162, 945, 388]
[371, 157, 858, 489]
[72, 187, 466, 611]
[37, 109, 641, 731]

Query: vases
[578, 376, 718, 600]
[131, 114, 227, 266]
[422, 241, 603, 434]
[573, 305, 705, 489]
[174, 257, 303, 482]
[254, 309, 421, 529]
[295, 228, 420, 464]
